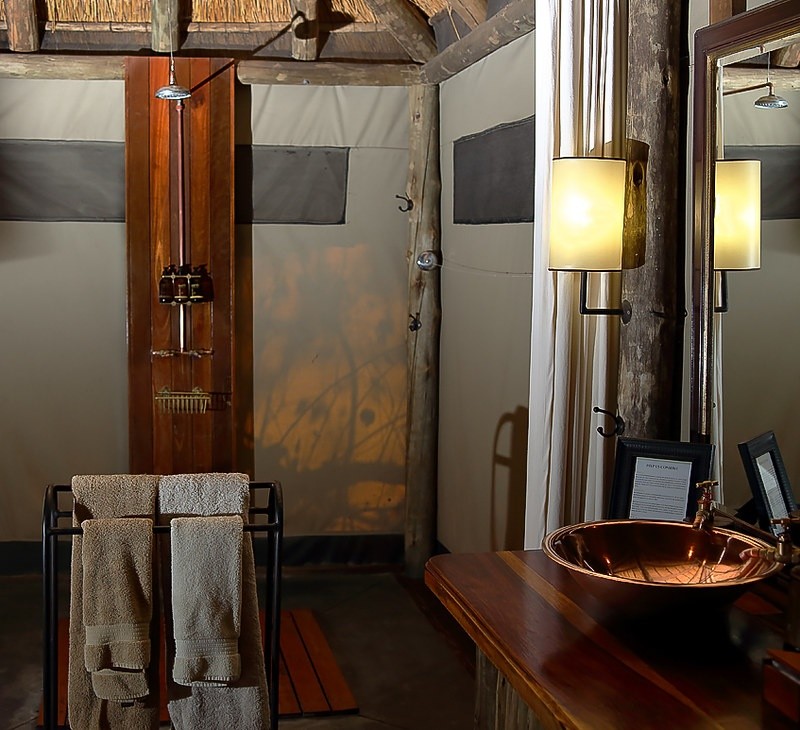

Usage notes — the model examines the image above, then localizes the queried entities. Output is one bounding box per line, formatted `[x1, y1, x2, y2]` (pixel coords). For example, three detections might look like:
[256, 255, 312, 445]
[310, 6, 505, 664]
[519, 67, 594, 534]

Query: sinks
[541, 519, 786, 616]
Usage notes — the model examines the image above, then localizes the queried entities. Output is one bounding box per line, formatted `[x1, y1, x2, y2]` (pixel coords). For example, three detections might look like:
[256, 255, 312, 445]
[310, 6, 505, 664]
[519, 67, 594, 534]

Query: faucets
[691, 480, 719, 533]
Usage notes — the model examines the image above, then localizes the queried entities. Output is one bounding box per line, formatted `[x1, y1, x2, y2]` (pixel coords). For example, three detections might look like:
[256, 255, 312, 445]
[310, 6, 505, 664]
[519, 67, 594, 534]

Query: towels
[61, 472, 273, 730]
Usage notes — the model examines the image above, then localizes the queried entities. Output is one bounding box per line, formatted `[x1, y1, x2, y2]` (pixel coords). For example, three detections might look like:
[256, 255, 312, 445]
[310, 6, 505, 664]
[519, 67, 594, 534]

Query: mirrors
[691, 0, 800, 562]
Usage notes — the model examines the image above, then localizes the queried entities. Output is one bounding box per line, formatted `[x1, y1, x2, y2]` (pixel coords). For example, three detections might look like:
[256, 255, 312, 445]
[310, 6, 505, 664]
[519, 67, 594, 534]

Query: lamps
[712, 160, 764, 315]
[549, 155, 632, 324]
